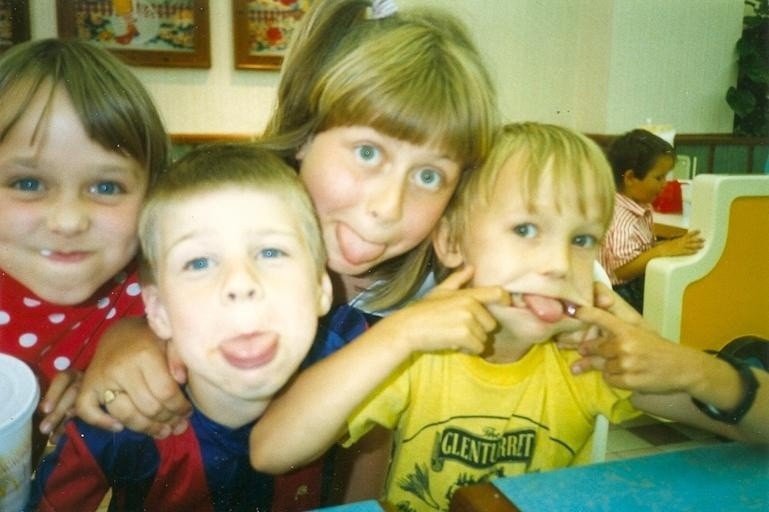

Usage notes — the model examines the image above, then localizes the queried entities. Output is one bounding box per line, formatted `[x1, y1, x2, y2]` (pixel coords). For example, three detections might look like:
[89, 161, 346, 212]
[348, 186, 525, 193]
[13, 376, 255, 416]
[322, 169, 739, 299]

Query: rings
[100, 388, 121, 407]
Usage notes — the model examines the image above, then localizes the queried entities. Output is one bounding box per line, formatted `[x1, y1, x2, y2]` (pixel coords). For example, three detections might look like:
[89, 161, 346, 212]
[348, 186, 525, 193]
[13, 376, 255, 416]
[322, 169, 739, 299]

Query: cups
[0, 352, 42, 512]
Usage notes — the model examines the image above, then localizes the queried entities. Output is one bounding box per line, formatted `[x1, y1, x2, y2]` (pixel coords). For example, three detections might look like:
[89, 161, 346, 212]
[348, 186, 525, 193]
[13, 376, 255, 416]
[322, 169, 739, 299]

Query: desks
[295, 493, 388, 511]
[442, 441, 765, 511]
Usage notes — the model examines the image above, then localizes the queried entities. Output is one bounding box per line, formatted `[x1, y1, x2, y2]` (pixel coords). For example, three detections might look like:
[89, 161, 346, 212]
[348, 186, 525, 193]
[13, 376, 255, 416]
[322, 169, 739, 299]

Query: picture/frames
[57, 3, 213, 68]
[233, 2, 341, 73]
[0, 0, 31, 48]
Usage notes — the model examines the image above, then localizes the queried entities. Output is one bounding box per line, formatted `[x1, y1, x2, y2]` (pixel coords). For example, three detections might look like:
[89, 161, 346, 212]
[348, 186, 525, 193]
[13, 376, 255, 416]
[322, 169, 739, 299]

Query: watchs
[692, 349, 761, 424]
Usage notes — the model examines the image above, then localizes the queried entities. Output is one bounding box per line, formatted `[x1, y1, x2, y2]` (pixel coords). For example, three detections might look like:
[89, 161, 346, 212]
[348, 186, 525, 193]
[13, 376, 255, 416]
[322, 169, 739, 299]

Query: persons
[596, 128, 705, 313]
[0, 39, 172, 446]
[248, 120, 768, 511]
[74, 0, 652, 440]
[23, 142, 395, 510]
